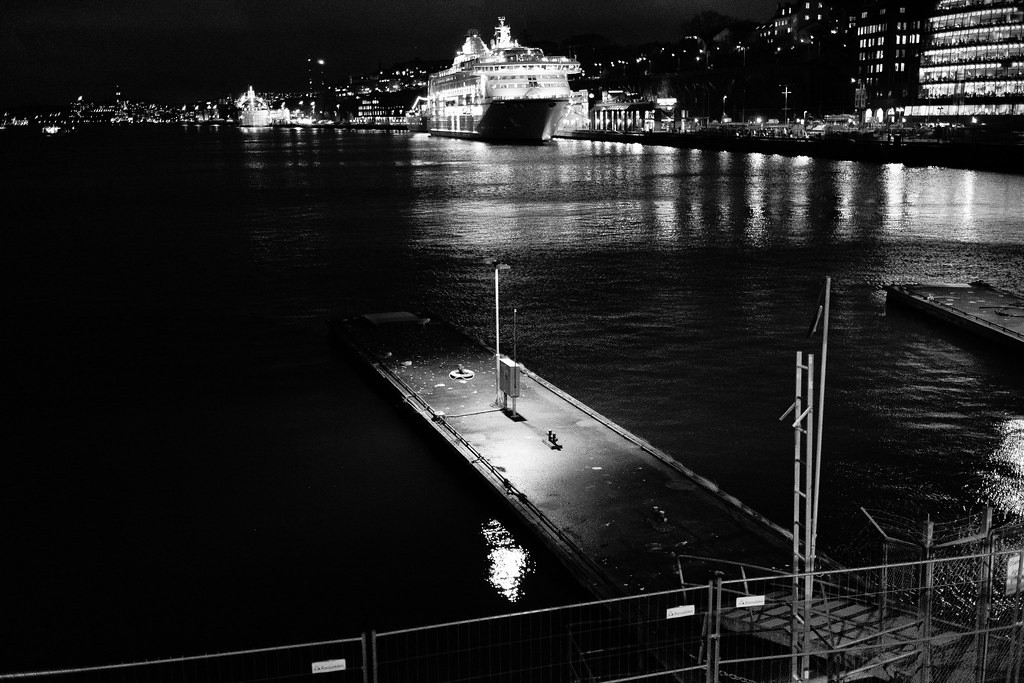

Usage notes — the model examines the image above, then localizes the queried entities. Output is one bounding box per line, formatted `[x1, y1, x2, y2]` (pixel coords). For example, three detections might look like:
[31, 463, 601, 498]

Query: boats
[406, 108, 427, 133]
[425, 16, 582, 146]
[444, 96, 458, 104]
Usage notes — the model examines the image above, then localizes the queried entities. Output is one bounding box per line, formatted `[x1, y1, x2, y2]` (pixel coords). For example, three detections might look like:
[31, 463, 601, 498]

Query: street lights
[495, 264, 510, 405]
